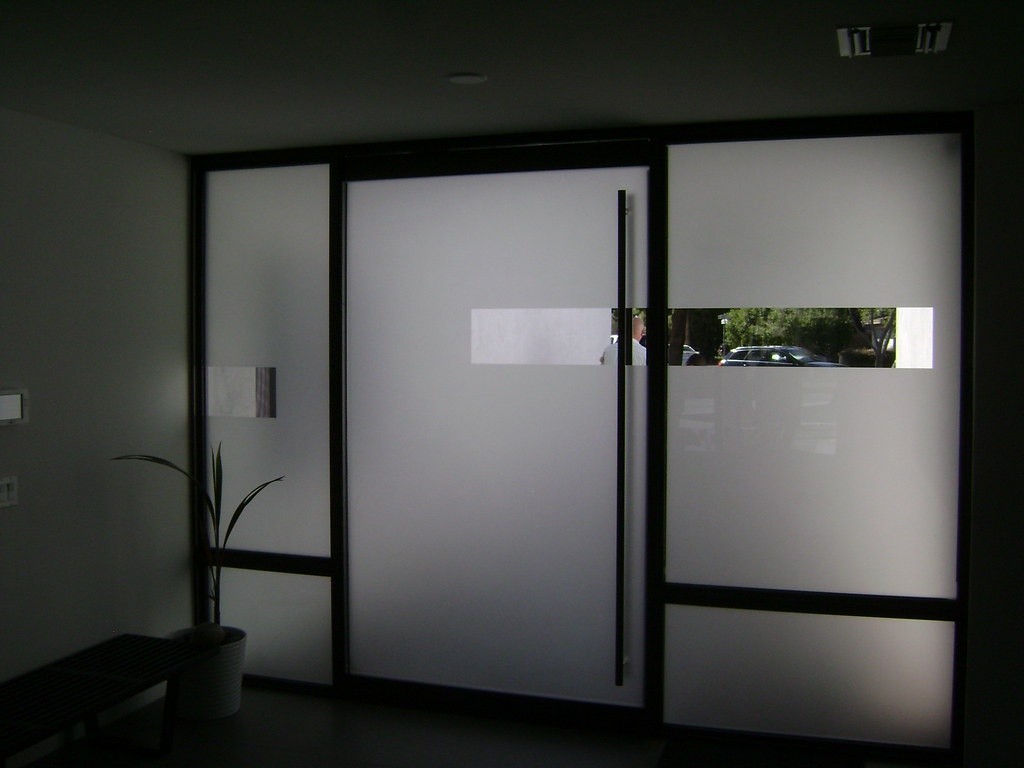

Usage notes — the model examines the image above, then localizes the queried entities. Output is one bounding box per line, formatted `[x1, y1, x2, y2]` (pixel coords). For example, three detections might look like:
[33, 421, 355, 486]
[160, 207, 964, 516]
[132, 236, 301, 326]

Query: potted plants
[107, 439, 286, 720]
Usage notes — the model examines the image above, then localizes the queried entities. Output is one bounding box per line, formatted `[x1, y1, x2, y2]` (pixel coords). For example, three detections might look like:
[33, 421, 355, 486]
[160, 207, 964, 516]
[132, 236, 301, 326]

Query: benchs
[0, 632, 221, 768]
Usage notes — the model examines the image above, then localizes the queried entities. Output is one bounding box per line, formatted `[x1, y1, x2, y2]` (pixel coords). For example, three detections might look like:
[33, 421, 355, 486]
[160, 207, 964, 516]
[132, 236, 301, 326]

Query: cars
[682, 344, 700, 365]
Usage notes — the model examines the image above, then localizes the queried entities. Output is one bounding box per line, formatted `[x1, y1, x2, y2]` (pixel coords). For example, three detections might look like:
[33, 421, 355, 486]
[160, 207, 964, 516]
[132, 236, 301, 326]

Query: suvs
[718, 345, 848, 367]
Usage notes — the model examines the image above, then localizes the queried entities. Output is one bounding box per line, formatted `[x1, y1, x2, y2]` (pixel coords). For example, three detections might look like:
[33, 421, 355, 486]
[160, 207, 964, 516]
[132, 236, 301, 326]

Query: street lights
[721, 319, 727, 357]
